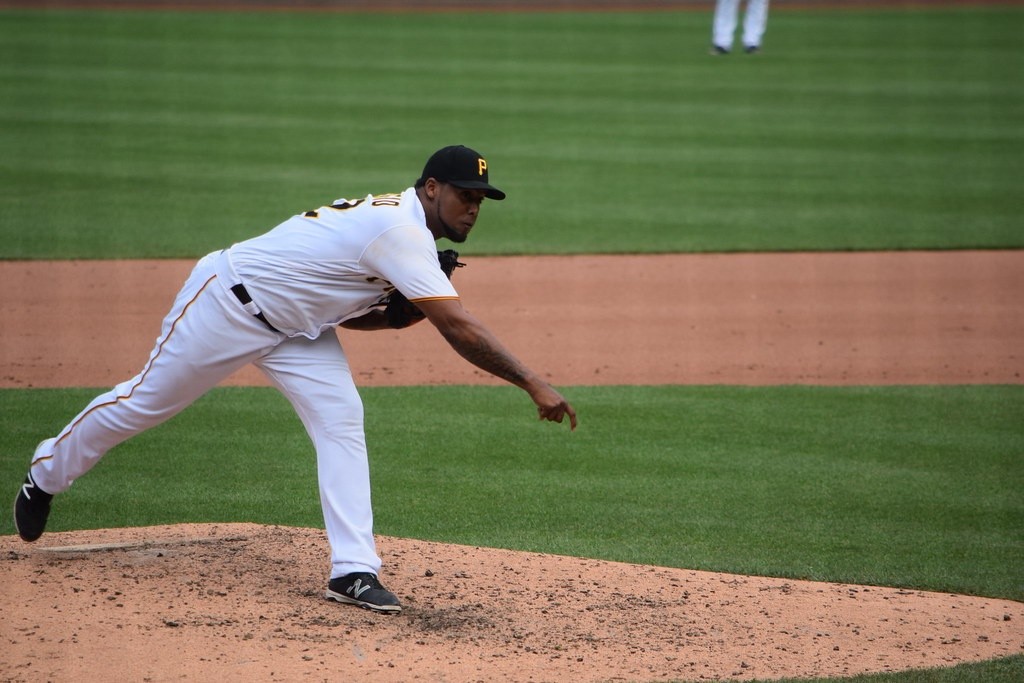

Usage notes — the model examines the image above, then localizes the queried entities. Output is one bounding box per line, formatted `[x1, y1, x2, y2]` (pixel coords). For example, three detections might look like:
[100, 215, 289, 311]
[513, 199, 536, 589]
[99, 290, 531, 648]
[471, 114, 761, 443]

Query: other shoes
[709, 44, 727, 56]
[742, 45, 758, 54]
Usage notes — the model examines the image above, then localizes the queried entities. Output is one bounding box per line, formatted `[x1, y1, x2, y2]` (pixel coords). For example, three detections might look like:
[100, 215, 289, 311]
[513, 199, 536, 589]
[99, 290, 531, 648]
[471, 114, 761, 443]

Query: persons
[13, 145, 577, 612]
[707, 0, 769, 56]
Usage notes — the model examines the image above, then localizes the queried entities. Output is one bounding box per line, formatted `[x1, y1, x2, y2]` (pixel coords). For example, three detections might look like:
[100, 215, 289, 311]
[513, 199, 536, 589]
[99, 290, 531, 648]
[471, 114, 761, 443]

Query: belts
[221, 248, 281, 333]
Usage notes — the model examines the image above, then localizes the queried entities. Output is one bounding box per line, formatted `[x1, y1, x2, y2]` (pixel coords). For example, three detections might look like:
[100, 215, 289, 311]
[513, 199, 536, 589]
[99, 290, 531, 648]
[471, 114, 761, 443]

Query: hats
[421, 145, 506, 200]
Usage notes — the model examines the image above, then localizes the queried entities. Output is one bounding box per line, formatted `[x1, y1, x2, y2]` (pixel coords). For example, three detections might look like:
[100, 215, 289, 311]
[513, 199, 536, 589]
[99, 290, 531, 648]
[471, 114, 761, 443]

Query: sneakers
[14, 439, 55, 542]
[324, 571, 402, 616]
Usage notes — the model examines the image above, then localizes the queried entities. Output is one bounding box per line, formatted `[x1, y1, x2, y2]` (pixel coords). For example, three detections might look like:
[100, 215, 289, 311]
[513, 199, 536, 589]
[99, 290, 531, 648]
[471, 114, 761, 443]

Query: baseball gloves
[380, 247, 459, 327]
